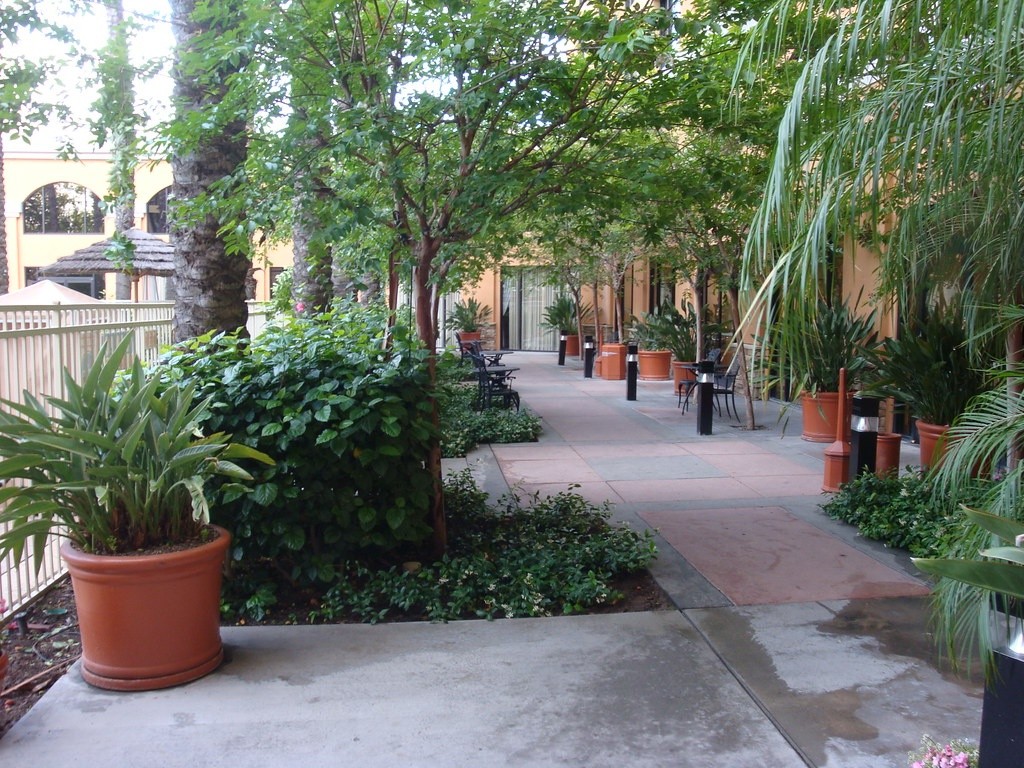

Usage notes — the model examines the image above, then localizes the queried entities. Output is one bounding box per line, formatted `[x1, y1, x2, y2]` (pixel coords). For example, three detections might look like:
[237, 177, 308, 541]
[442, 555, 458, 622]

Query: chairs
[681, 348, 741, 424]
[455, 333, 520, 415]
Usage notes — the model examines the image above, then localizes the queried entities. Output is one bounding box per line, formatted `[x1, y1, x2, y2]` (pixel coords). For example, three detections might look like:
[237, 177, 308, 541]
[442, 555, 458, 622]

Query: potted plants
[851, 300, 992, 479]
[0, 329, 278, 679]
[621, 296, 734, 397]
[746, 284, 894, 444]
[538, 292, 604, 355]
[441, 297, 494, 351]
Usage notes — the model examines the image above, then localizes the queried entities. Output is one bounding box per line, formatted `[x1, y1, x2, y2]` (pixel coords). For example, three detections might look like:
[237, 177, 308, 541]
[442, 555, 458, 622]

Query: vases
[877, 432, 901, 480]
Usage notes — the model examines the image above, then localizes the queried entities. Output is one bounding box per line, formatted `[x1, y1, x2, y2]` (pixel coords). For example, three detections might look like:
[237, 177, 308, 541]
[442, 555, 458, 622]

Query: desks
[682, 366, 727, 411]
[462, 340, 486, 354]
[480, 351, 513, 367]
[474, 368, 520, 391]
